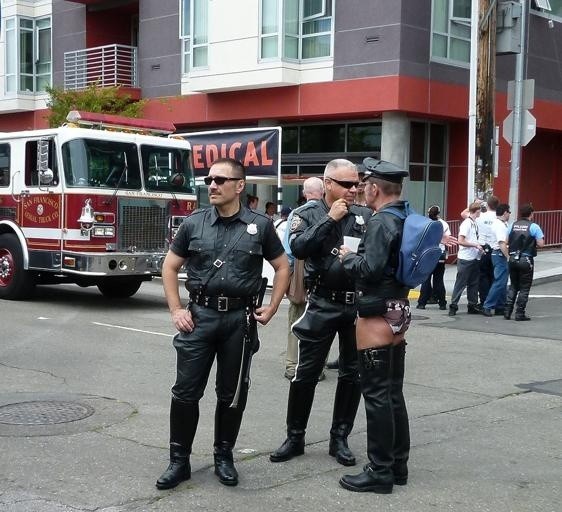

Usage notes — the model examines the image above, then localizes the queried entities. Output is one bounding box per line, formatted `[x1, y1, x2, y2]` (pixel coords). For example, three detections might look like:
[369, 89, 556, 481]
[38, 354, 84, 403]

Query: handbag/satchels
[358, 295, 388, 320]
[285, 257, 306, 306]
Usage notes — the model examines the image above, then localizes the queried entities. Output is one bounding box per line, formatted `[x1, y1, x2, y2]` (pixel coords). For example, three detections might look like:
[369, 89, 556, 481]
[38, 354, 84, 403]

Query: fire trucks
[0, 110, 198, 305]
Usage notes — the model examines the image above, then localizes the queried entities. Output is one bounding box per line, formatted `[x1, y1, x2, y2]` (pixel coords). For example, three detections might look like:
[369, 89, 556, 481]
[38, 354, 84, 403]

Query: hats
[428, 205, 441, 213]
[356, 163, 366, 174]
[281, 207, 293, 215]
[361, 156, 410, 184]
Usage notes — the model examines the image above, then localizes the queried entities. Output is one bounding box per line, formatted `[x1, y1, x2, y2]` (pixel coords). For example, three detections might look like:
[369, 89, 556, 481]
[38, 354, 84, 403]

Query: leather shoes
[416, 302, 426, 309]
[515, 316, 532, 321]
[448, 303, 459, 316]
[438, 302, 447, 310]
[504, 314, 512, 319]
[494, 307, 504, 315]
[327, 354, 340, 370]
[467, 304, 493, 318]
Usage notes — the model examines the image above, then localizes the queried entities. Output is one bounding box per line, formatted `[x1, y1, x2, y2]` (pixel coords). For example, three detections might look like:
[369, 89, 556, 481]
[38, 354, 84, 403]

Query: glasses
[326, 176, 360, 190]
[204, 174, 243, 186]
[504, 209, 512, 215]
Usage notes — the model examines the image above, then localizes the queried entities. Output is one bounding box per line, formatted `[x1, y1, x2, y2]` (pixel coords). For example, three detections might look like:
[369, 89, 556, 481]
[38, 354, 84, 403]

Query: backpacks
[378, 200, 444, 290]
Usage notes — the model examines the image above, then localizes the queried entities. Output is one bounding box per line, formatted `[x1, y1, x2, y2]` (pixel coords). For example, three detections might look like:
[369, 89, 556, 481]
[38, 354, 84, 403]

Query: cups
[343, 235, 361, 254]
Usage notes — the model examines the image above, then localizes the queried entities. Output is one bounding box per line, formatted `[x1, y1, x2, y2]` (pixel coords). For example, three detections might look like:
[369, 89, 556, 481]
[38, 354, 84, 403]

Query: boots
[328, 381, 362, 467]
[269, 380, 318, 463]
[338, 339, 411, 495]
[153, 392, 201, 491]
[212, 390, 247, 488]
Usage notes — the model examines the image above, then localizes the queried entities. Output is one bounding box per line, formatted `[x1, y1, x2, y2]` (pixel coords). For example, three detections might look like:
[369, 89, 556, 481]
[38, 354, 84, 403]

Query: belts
[187, 290, 257, 314]
[310, 283, 357, 306]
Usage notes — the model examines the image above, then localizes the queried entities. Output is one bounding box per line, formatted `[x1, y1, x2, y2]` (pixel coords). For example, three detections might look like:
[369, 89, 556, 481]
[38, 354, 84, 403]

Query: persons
[282, 176, 328, 384]
[270, 157, 375, 466]
[326, 163, 370, 370]
[153, 156, 292, 490]
[337, 153, 413, 494]
[247, 194, 546, 324]
